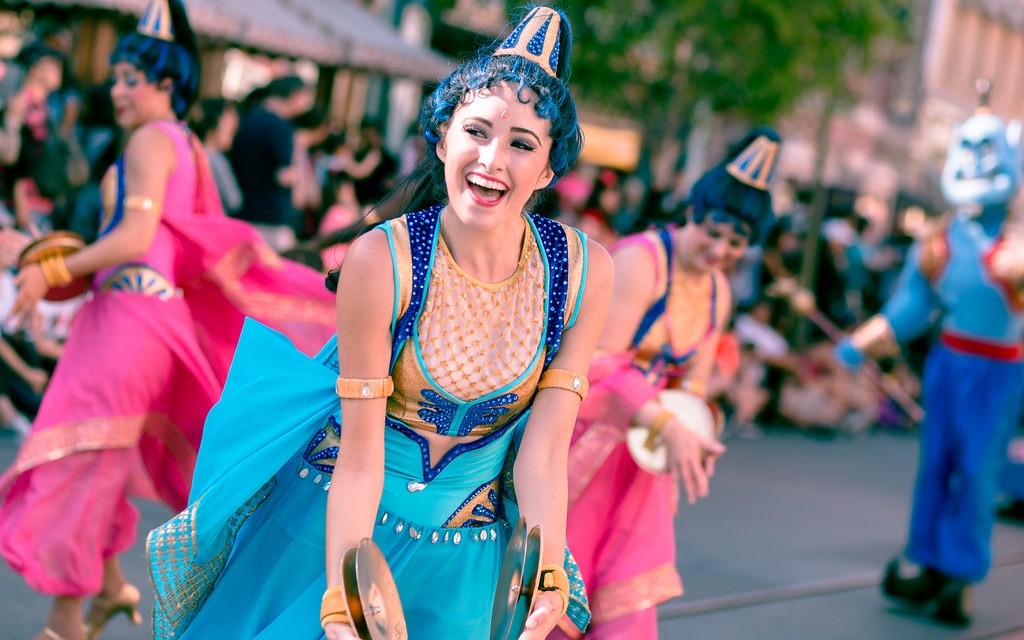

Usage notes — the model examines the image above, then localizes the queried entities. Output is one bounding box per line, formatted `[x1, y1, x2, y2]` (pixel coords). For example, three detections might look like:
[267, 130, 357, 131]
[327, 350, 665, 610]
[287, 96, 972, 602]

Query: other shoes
[930, 583, 973, 629]
[880, 555, 942, 607]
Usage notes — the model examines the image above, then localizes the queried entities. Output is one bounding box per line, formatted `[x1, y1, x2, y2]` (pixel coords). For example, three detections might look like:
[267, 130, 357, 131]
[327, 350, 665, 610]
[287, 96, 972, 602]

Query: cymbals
[338, 536, 412, 640]
[627, 391, 725, 477]
[487, 517, 545, 640]
[17, 229, 91, 302]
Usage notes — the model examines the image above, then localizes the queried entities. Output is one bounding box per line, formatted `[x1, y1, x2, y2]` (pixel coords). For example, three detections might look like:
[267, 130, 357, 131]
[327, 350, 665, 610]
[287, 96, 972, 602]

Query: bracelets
[644, 409, 677, 451]
[39, 254, 73, 288]
[318, 587, 351, 631]
[538, 563, 569, 619]
[831, 337, 870, 370]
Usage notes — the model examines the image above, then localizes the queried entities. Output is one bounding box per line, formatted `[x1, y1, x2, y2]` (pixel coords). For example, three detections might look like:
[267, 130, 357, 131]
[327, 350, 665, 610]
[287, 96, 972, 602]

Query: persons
[3, 14, 432, 439]
[536, 159, 805, 435]
[807, 109, 1024, 625]
[543, 127, 783, 640]
[1, 0, 210, 639]
[144, 6, 617, 640]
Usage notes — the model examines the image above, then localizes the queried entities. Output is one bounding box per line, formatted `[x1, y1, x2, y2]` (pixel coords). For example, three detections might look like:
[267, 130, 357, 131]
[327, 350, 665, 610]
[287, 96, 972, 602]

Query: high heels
[86, 584, 142, 640]
[44, 625, 90, 640]
